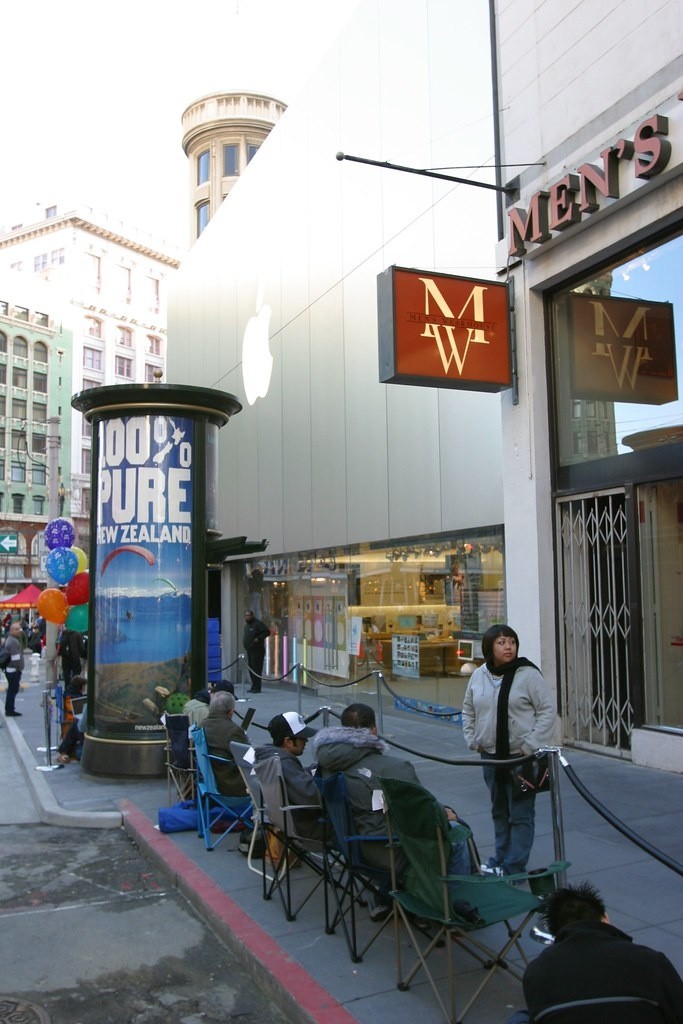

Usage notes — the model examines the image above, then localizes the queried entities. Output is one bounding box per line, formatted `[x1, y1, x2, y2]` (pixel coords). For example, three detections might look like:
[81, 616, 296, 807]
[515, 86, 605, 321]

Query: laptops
[241, 708, 256, 735]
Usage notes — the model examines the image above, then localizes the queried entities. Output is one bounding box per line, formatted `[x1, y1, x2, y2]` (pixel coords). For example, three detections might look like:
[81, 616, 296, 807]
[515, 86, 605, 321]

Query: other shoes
[5, 711, 22, 717]
[246, 689, 261, 693]
[480, 857, 523, 882]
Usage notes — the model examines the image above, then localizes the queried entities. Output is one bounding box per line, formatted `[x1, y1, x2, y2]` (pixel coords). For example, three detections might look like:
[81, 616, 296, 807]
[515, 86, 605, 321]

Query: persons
[505, 884, 683, 1024]
[243, 610, 271, 693]
[462, 624, 554, 883]
[253, 712, 397, 921]
[0, 611, 46, 716]
[314, 703, 470, 940]
[43, 628, 87, 765]
[248, 563, 264, 621]
[183, 680, 238, 726]
[198, 692, 265, 859]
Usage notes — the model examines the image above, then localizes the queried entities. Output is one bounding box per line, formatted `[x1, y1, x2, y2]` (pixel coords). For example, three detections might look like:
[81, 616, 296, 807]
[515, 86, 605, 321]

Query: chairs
[163, 711, 570, 1024]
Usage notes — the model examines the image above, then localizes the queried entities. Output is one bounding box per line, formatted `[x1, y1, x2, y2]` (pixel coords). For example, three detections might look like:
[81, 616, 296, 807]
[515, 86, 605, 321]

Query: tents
[0, 583, 42, 634]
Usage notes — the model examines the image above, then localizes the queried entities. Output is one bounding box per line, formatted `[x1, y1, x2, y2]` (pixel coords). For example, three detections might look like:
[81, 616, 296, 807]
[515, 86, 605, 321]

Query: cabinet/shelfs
[383, 637, 456, 679]
[461, 588, 507, 634]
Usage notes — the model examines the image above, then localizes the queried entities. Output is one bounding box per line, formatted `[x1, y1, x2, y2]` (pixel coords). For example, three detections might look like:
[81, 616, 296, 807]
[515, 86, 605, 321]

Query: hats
[212, 679, 239, 700]
[268, 712, 320, 745]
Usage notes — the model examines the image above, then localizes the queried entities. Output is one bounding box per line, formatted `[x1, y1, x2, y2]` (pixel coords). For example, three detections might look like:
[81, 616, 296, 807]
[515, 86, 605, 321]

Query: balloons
[37, 518, 89, 635]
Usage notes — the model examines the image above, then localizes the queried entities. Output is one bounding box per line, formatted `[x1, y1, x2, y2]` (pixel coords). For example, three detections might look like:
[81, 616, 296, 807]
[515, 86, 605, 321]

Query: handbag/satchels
[508, 751, 554, 799]
[0, 637, 12, 670]
[68, 649, 82, 675]
